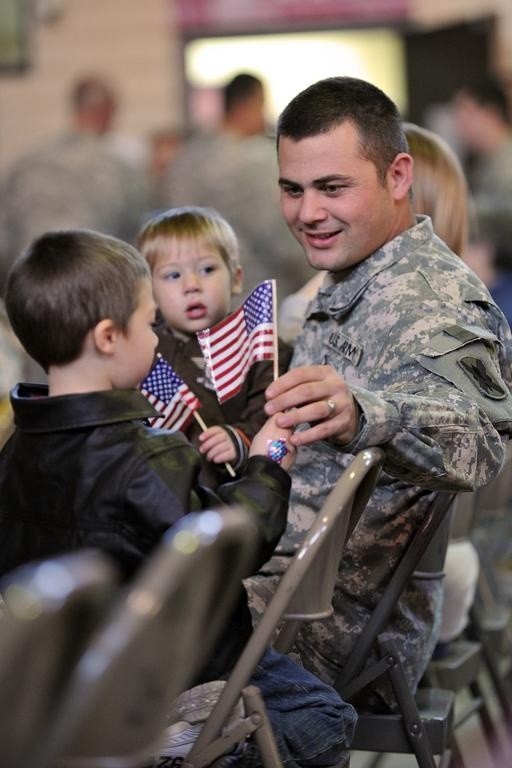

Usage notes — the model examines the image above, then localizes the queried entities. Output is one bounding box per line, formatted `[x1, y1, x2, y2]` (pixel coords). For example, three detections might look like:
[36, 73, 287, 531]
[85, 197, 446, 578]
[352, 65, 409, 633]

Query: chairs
[1, 444, 512, 767]
